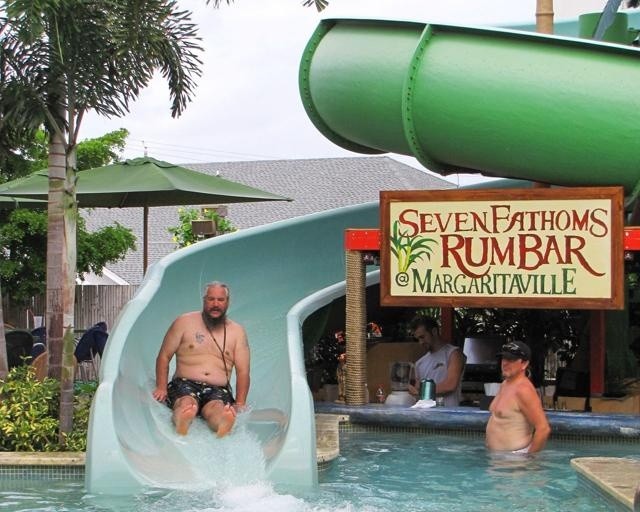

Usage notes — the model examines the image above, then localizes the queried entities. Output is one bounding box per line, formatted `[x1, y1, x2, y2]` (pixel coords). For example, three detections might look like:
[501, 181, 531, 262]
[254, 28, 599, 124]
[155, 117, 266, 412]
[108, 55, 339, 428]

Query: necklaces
[205, 325, 230, 392]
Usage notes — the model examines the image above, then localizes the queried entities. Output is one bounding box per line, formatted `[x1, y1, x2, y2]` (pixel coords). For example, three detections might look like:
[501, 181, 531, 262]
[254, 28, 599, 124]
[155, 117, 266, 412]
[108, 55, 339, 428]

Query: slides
[83, 12, 640, 495]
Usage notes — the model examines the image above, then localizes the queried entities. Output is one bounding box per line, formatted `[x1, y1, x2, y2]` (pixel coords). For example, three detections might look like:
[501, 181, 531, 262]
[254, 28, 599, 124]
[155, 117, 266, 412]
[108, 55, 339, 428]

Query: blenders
[385, 360, 418, 408]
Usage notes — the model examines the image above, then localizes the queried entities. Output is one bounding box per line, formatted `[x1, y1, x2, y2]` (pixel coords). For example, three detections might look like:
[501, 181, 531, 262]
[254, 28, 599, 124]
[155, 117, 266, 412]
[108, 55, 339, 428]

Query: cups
[541, 398, 559, 410]
[435, 395, 446, 407]
[561, 400, 566, 409]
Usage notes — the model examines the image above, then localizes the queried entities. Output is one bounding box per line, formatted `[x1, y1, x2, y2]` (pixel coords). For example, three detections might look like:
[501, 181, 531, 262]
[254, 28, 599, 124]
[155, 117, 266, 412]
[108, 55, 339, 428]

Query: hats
[495, 340, 532, 361]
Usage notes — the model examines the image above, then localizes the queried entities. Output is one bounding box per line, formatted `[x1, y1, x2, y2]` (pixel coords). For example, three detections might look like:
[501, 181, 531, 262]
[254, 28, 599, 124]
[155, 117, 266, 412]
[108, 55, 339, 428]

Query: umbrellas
[0, 167, 67, 212]
[1, 146, 295, 282]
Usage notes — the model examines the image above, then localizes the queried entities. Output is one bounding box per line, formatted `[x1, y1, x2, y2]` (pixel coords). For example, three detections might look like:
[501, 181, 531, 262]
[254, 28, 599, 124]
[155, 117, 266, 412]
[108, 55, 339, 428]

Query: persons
[151, 279, 251, 439]
[483, 341, 552, 479]
[406, 313, 466, 407]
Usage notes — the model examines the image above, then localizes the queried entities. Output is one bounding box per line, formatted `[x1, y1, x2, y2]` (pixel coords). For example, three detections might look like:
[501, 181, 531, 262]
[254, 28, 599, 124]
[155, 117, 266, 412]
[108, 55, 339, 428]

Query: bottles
[419, 378, 436, 402]
[376, 384, 385, 403]
[364, 384, 370, 403]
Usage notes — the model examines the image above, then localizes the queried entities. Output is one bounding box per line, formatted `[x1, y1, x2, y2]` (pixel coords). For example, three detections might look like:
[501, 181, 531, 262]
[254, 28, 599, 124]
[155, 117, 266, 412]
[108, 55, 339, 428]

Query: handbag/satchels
[553, 367, 593, 413]
